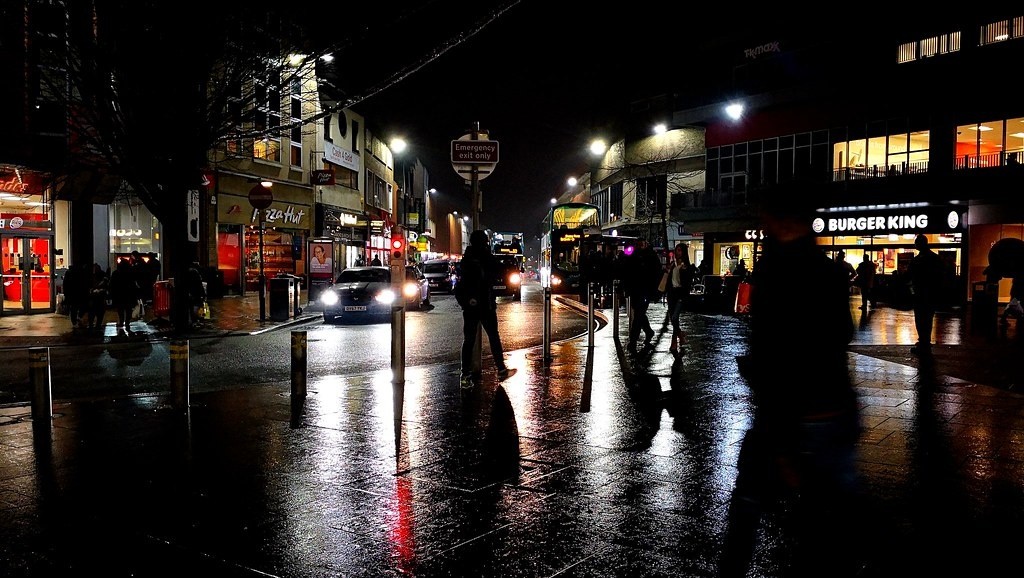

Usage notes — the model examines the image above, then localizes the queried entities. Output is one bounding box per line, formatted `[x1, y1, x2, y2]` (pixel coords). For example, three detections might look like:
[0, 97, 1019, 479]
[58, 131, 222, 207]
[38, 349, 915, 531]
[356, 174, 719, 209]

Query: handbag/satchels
[658, 262, 674, 292]
[131, 300, 145, 318]
[454, 279, 484, 310]
[197, 302, 210, 319]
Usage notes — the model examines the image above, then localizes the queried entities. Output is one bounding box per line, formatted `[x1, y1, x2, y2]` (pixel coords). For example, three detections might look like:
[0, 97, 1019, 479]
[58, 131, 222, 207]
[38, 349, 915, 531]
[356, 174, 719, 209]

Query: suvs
[419, 259, 457, 290]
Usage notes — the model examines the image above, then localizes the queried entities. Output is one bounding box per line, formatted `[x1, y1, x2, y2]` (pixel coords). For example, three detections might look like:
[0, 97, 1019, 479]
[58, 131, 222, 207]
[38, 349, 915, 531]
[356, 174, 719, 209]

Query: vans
[493, 254, 525, 301]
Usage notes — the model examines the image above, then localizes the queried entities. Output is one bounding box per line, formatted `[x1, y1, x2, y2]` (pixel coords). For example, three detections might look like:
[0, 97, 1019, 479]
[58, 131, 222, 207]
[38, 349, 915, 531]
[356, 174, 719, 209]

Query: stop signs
[248, 184, 273, 210]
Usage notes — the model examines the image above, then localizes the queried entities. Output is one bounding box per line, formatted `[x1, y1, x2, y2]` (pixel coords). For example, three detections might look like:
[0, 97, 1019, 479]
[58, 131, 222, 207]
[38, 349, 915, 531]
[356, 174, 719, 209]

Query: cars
[405, 266, 431, 309]
[323, 265, 391, 322]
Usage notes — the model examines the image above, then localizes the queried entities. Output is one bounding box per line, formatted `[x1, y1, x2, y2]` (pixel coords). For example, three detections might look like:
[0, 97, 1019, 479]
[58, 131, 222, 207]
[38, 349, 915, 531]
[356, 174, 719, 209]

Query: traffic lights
[390, 235, 406, 266]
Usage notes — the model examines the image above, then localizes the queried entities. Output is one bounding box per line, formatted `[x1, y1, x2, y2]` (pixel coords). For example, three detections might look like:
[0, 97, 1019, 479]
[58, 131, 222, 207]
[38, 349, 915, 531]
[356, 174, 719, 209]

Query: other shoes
[647, 329, 655, 339]
[911, 344, 931, 356]
[498, 369, 516, 382]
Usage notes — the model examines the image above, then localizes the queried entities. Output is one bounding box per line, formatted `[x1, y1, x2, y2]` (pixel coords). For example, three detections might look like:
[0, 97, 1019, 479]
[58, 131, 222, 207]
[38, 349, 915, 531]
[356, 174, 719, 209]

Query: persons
[354, 254, 364, 267]
[733, 259, 747, 275]
[856, 254, 876, 310]
[1002, 297, 1024, 323]
[833, 250, 857, 303]
[310, 245, 332, 272]
[370, 255, 382, 266]
[666, 243, 692, 349]
[30, 247, 41, 267]
[719, 197, 860, 578]
[459, 231, 517, 390]
[618, 237, 663, 345]
[903, 235, 946, 359]
[511, 237, 519, 246]
[63, 250, 161, 331]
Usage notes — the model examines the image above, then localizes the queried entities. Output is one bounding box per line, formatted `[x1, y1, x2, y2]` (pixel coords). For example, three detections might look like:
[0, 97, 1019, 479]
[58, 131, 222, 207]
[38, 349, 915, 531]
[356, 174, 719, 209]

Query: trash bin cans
[720, 276, 742, 314]
[701, 276, 721, 314]
[269, 278, 295, 321]
[277, 273, 303, 315]
[970, 281, 999, 338]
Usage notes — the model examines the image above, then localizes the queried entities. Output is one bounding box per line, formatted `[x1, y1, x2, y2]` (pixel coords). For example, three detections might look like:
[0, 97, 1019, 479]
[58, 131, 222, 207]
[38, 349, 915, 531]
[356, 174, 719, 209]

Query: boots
[670, 335, 678, 352]
[679, 332, 690, 346]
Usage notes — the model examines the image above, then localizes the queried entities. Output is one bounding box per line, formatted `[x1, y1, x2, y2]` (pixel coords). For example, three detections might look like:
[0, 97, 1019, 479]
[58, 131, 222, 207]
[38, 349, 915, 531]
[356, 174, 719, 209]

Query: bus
[540, 203, 602, 294]
[490, 232, 525, 254]
[579, 234, 639, 308]
